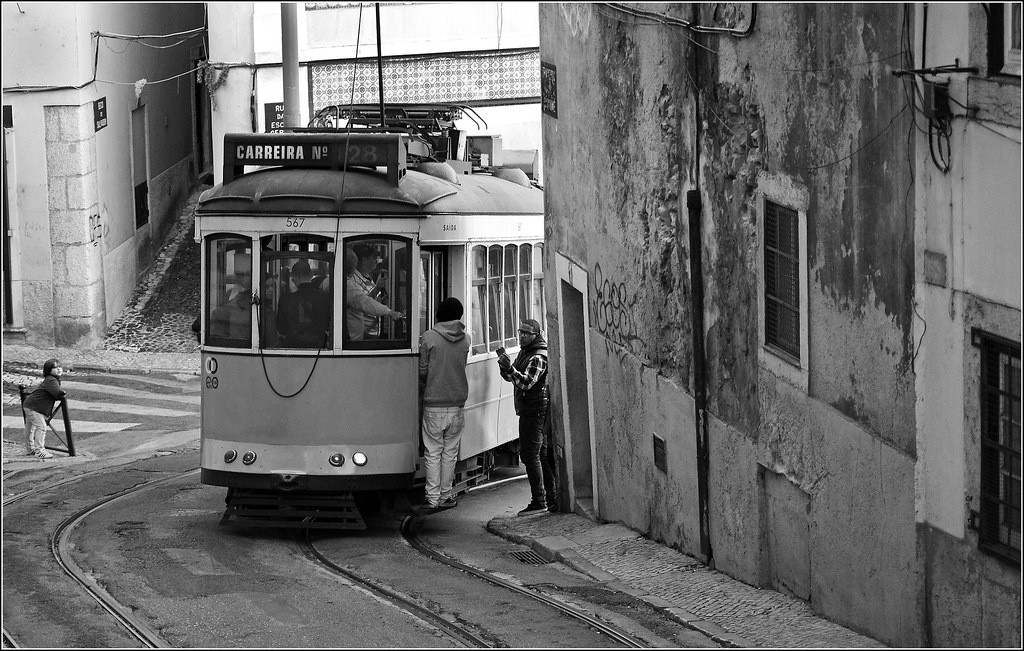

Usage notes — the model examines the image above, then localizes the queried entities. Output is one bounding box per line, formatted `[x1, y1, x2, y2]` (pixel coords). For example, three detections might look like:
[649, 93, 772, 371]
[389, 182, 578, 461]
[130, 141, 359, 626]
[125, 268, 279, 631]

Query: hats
[436, 298, 463, 322]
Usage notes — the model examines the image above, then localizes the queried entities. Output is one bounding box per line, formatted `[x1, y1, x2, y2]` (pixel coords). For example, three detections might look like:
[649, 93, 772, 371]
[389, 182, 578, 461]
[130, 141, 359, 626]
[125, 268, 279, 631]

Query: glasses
[518, 329, 537, 338]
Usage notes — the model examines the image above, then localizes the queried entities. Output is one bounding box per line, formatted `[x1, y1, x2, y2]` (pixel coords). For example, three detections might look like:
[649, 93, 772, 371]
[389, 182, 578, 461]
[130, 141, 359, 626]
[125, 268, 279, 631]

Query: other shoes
[439, 498, 456, 507]
[420, 499, 435, 509]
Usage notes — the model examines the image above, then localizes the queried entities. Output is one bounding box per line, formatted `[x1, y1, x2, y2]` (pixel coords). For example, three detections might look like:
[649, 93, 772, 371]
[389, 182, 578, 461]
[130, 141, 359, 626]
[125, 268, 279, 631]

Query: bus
[192, 101, 551, 534]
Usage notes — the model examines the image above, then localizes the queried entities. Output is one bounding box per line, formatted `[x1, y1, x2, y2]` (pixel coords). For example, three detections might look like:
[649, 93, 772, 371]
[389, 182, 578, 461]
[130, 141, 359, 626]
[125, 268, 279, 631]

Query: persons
[24, 359, 67, 458]
[497, 318, 562, 516]
[418, 297, 469, 509]
[213, 243, 408, 353]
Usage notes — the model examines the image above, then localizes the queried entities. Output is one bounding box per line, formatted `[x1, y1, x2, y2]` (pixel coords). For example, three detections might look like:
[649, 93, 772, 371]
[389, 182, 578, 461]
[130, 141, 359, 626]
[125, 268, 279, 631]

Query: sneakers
[29, 451, 35, 455]
[35, 451, 53, 459]
[518, 503, 548, 515]
